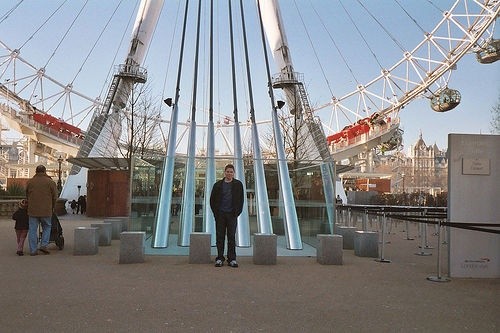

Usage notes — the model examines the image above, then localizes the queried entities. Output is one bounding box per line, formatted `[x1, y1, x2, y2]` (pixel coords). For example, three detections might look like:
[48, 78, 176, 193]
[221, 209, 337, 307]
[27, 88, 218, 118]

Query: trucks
[343, 175, 392, 194]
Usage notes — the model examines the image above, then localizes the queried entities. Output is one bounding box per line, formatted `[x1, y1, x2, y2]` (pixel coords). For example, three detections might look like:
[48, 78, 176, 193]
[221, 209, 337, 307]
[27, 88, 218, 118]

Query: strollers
[36, 204, 65, 250]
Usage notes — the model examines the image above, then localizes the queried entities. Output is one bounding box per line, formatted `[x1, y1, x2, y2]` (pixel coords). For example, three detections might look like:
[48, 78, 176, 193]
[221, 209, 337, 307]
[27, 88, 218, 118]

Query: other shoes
[227, 259, 238, 267]
[39, 246, 50, 254]
[215, 258, 223, 267]
[17, 250, 23, 255]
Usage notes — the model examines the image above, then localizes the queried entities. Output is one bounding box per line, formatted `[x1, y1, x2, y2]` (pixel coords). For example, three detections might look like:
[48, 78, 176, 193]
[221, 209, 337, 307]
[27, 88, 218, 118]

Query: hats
[18, 199, 28, 209]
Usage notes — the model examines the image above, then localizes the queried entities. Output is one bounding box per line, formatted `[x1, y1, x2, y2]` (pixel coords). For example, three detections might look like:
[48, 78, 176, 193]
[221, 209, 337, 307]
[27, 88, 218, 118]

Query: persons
[335, 195, 343, 217]
[210, 164, 244, 268]
[68, 195, 87, 215]
[12, 165, 58, 256]
